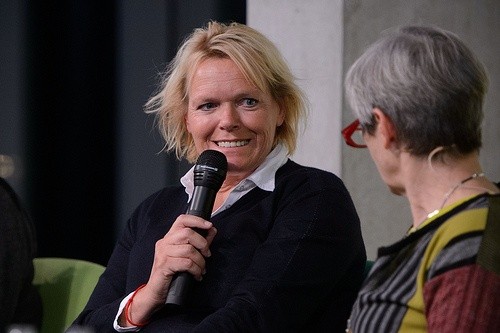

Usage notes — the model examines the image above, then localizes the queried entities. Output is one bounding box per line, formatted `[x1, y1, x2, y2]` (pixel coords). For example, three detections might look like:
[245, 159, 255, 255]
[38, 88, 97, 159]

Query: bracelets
[126, 283, 148, 326]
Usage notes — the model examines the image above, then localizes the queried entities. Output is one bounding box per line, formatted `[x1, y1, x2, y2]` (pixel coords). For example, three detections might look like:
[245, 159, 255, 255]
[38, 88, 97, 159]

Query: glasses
[341, 119, 367, 148]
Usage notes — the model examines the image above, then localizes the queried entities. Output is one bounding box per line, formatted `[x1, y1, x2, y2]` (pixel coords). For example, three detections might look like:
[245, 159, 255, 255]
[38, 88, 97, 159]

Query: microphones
[166, 149, 227, 306]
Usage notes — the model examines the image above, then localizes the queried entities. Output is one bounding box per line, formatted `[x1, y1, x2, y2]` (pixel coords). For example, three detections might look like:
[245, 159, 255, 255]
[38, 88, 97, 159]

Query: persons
[341, 25, 500, 332]
[62, 22, 367, 333]
[0, 177, 36, 333]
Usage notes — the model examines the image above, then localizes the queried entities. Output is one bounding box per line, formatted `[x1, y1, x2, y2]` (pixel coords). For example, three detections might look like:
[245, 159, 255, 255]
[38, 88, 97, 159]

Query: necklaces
[430, 174, 483, 215]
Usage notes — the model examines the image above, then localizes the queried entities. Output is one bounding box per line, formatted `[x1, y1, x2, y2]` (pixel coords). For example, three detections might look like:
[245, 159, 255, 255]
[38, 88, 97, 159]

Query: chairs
[32, 258, 106, 333]
